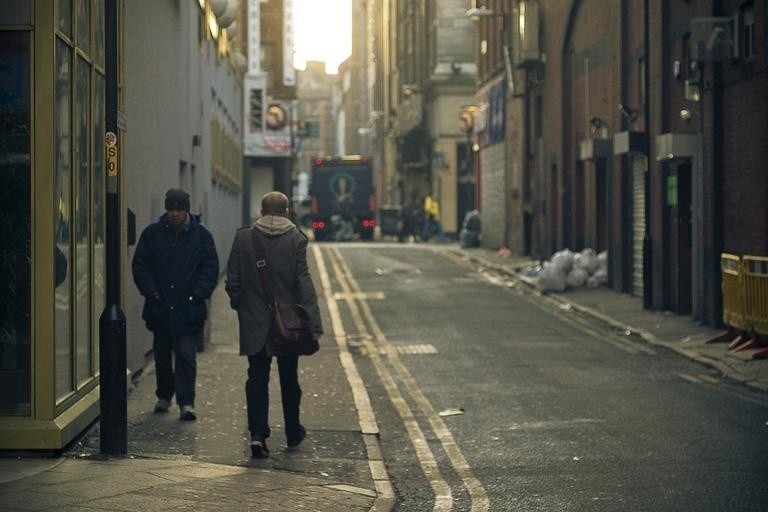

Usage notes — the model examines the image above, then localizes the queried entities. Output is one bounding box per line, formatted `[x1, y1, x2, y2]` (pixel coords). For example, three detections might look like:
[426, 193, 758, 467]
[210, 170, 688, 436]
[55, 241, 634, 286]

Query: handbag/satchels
[269, 296, 319, 354]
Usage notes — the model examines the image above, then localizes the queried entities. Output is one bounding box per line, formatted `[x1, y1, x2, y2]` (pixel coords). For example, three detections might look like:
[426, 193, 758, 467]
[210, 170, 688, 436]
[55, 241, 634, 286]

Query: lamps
[465, 8, 503, 21]
[684, 78, 703, 103]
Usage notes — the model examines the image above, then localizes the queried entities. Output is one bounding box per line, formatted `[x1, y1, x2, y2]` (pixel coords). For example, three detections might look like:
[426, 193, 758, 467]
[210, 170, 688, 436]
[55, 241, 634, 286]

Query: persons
[417, 191, 441, 240]
[129, 186, 222, 421]
[219, 190, 326, 459]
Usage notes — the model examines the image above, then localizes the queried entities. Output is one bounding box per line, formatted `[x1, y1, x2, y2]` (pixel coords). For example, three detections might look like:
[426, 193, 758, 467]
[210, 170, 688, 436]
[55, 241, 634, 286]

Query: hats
[164, 188, 189, 211]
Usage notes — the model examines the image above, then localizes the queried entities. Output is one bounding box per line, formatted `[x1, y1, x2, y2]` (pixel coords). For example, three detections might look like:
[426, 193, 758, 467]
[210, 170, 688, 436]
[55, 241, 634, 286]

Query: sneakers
[287, 424, 305, 450]
[180, 405, 196, 419]
[155, 399, 171, 411]
[250, 434, 269, 458]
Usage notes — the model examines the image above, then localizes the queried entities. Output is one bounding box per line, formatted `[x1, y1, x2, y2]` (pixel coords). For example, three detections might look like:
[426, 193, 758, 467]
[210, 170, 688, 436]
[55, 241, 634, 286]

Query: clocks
[265, 104, 286, 129]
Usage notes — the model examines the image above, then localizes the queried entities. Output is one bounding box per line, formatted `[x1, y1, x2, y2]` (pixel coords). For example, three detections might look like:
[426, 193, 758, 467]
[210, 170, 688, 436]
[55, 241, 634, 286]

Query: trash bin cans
[381, 208, 400, 241]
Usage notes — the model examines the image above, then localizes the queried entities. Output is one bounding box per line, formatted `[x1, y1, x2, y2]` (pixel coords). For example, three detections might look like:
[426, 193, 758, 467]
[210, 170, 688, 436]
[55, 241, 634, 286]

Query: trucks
[308, 154, 376, 244]
